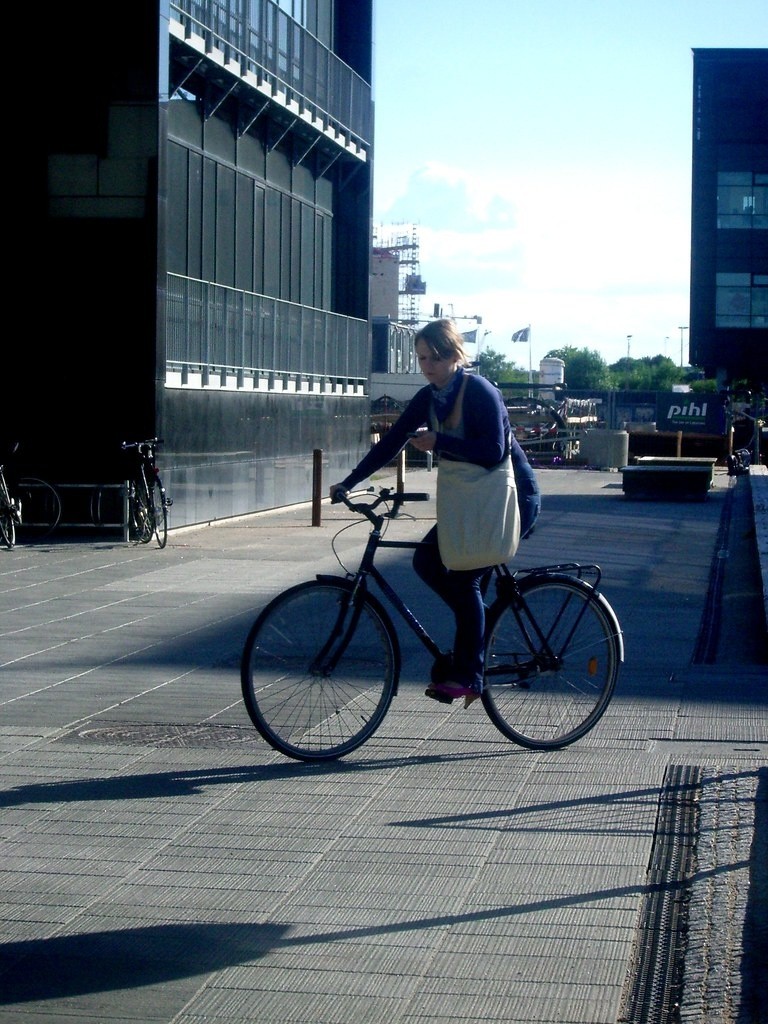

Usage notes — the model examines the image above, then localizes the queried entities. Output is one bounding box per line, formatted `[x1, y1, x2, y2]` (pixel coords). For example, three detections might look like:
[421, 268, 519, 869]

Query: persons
[330, 318, 540, 709]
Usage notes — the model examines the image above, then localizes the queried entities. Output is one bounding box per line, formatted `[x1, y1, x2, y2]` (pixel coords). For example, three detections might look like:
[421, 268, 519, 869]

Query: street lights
[678, 326, 689, 368]
[627, 334, 633, 357]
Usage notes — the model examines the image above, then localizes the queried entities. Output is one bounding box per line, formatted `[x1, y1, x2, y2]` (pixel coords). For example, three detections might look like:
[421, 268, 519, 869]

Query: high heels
[427, 682, 483, 710]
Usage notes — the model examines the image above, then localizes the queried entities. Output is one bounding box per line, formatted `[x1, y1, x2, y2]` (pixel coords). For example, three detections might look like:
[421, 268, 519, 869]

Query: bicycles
[241, 487, 625, 762]
[121, 437, 174, 549]
[0, 440, 63, 550]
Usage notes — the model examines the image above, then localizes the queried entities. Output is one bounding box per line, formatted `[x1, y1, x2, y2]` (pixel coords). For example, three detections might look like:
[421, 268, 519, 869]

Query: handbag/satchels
[436, 445, 522, 571]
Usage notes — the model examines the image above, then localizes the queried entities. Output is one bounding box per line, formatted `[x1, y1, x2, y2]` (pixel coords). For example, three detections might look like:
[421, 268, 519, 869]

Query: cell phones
[407, 433, 420, 439]
